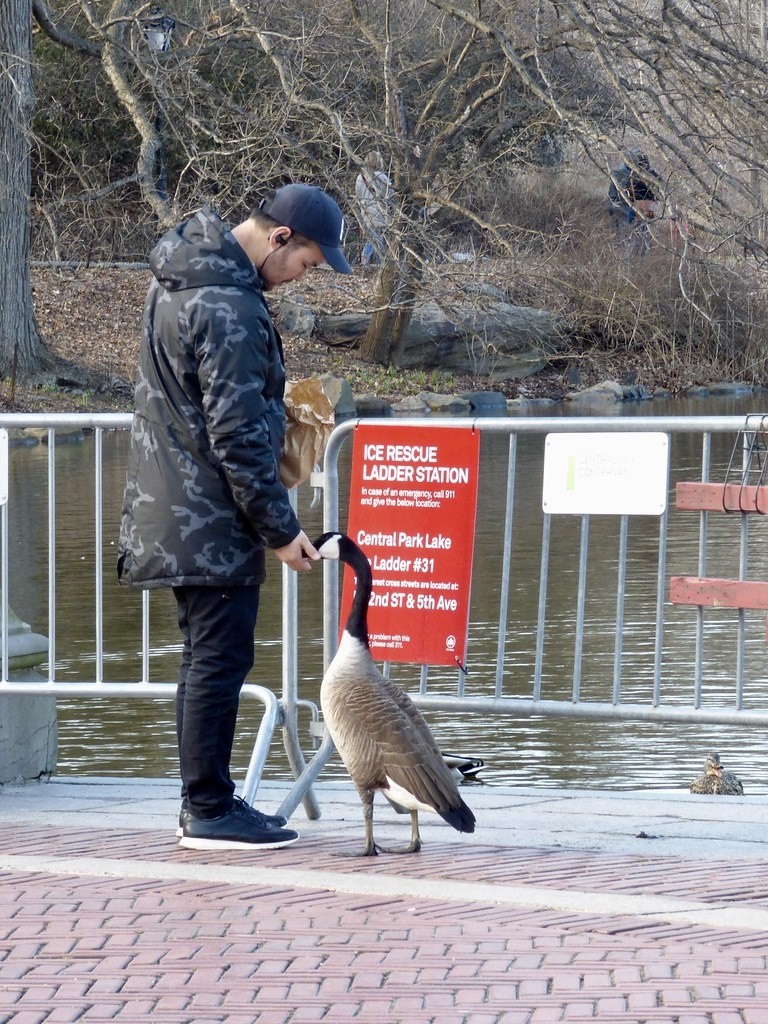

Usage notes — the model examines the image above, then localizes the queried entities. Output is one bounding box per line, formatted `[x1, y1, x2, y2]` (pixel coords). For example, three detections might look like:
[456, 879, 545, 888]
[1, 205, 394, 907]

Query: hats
[259, 184, 352, 274]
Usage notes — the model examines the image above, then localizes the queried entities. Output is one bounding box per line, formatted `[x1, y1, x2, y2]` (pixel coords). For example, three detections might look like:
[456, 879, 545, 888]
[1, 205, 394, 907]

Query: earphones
[275, 235, 288, 246]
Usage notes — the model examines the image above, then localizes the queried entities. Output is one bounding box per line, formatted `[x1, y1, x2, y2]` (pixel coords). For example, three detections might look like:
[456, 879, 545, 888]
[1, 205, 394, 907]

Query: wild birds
[301, 531, 478, 858]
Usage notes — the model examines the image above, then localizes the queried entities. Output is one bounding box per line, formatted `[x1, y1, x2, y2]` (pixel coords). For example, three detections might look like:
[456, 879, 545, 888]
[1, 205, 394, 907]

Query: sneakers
[179, 813, 299, 851]
[176, 795, 288, 838]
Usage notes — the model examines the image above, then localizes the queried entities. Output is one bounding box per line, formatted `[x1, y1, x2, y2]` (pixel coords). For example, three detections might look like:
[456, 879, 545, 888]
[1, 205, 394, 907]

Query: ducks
[690, 753, 743, 795]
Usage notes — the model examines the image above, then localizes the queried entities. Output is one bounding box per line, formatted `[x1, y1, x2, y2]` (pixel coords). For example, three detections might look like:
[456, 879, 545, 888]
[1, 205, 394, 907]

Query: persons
[356, 150, 398, 266]
[607, 150, 665, 214]
[108, 181, 355, 855]
